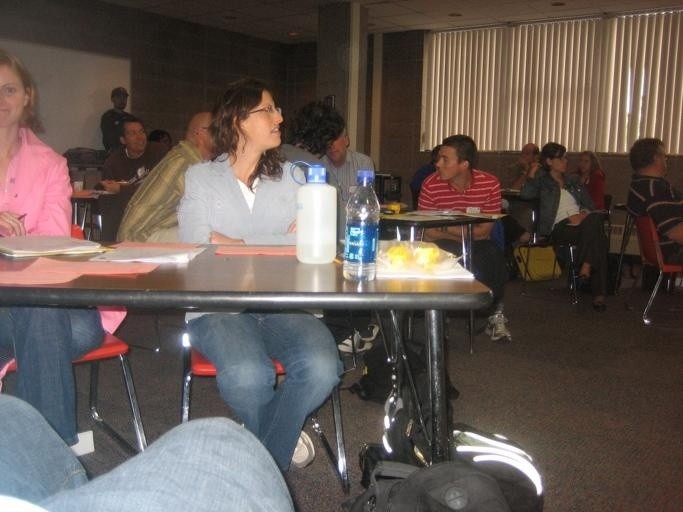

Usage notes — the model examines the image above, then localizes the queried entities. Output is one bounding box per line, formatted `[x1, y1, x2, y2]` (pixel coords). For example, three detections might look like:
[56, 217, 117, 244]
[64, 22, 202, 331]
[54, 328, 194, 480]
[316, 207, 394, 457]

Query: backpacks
[339, 460, 510, 512]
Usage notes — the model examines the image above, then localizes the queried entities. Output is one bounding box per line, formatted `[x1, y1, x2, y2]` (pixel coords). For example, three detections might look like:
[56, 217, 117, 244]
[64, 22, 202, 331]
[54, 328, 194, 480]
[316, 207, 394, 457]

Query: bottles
[343, 171, 379, 283]
[289, 160, 337, 262]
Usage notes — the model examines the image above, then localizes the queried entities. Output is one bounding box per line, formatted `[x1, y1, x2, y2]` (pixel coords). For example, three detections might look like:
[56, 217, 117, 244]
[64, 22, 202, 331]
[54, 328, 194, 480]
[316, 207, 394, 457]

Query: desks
[66, 187, 144, 245]
[0, 234, 494, 476]
[67, 160, 105, 193]
[368, 200, 509, 361]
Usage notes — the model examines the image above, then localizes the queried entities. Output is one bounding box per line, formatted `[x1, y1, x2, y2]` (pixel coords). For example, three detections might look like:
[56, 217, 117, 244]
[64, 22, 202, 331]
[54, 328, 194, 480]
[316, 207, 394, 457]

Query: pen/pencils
[17, 213, 28, 221]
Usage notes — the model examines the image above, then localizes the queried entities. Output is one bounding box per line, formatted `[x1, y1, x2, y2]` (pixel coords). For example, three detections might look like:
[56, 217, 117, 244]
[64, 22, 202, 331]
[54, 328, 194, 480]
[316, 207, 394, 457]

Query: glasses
[246, 104, 283, 114]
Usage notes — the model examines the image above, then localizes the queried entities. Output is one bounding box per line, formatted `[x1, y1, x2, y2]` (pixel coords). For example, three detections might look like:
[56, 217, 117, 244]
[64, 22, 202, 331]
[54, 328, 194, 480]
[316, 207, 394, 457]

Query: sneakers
[291, 430, 316, 468]
[480, 310, 512, 343]
[356, 323, 379, 342]
[337, 332, 373, 353]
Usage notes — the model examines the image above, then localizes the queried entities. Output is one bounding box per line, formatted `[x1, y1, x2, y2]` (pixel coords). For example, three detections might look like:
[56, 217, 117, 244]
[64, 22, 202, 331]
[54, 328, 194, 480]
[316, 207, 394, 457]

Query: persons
[626, 137, 683, 273]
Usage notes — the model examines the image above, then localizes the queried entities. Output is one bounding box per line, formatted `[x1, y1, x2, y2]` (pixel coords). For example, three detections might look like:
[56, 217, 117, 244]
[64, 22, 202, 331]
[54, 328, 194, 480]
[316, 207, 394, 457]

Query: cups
[384, 179, 400, 213]
[73, 181, 83, 194]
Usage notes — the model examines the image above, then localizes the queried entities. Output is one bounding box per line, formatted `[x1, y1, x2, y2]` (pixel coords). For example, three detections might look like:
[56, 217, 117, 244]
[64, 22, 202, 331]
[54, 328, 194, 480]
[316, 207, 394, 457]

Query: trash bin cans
[92, 214, 103, 241]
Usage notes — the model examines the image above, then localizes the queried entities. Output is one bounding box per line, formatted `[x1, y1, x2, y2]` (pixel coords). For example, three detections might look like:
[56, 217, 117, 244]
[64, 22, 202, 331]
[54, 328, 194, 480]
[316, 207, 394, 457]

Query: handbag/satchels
[512, 245, 561, 281]
[358, 393, 542, 512]
[348, 339, 459, 404]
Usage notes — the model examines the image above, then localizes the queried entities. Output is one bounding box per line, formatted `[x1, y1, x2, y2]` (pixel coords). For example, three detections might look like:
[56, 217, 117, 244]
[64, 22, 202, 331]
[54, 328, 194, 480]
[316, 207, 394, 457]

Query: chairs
[493, 187, 682, 326]
[169, 301, 356, 497]
[1, 324, 152, 465]
